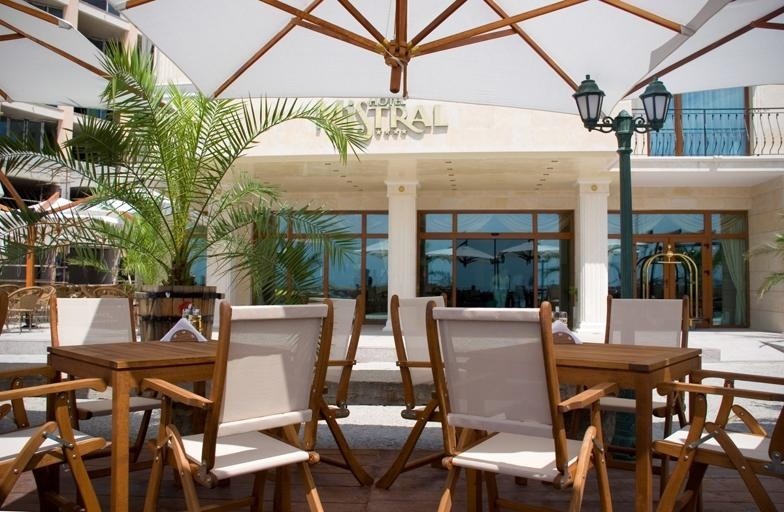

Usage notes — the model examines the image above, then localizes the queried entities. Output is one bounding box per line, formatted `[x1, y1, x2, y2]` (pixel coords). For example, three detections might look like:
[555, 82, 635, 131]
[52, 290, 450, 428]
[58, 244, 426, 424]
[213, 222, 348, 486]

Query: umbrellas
[623, 2, 784, 97]
[61, 192, 213, 244]
[100, 2, 715, 126]
[0, 0, 118, 109]
[0, 194, 129, 257]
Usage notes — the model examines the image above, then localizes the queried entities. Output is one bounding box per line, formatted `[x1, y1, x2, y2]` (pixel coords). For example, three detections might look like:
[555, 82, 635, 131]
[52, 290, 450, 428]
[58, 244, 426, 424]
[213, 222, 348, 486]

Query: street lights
[573, 73, 672, 461]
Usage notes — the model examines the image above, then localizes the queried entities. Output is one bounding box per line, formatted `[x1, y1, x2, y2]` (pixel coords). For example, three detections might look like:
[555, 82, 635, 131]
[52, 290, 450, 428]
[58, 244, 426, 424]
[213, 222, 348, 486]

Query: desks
[549, 330, 706, 512]
[44, 341, 220, 511]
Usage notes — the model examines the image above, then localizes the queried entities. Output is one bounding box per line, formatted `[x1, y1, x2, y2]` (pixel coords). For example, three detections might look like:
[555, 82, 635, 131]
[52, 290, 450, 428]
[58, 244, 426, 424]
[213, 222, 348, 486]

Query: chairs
[0, 366, 112, 511]
[143, 295, 336, 511]
[651, 369, 783, 511]
[44, 290, 162, 480]
[265, 292, 375, 490]
[566, 291, 696, 497]
[426, 298, 620, 512]
[376, 293, 461, 494]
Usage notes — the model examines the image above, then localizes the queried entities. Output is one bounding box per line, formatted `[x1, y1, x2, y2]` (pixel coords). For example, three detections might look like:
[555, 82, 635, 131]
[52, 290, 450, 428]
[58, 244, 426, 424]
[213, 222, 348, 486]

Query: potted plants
[0, 35, 371, 342]
